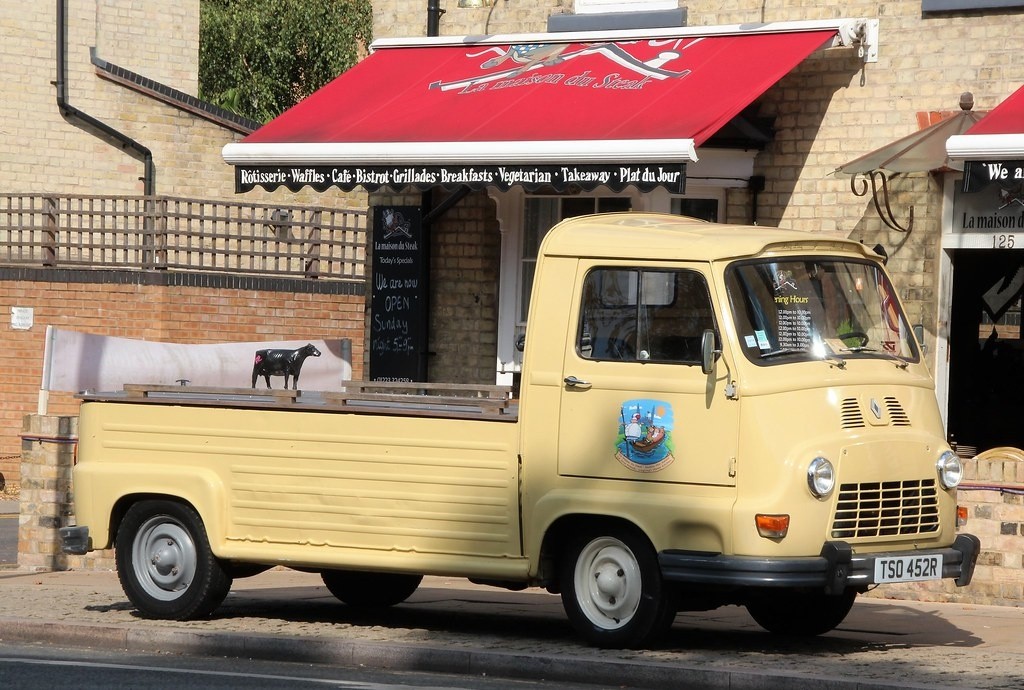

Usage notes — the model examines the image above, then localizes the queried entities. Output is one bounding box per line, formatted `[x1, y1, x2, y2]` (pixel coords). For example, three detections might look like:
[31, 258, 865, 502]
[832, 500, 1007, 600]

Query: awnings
[221, 21, 845, 192]
[828, 92, 990, 176]
[944, 82, 1023, 163]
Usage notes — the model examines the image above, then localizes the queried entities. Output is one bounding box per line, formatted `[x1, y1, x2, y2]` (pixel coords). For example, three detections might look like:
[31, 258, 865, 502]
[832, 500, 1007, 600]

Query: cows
[251, 343, 321, 391]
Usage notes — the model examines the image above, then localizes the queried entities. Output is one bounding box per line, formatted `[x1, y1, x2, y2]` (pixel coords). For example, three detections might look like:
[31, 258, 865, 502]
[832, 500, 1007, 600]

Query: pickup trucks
[56, 208, 982, 623]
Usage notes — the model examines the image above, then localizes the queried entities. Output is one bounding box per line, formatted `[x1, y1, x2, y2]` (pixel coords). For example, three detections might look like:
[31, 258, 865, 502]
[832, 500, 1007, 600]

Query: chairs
[591, 337, 636, 361]
[663, 335, 722, 363]
